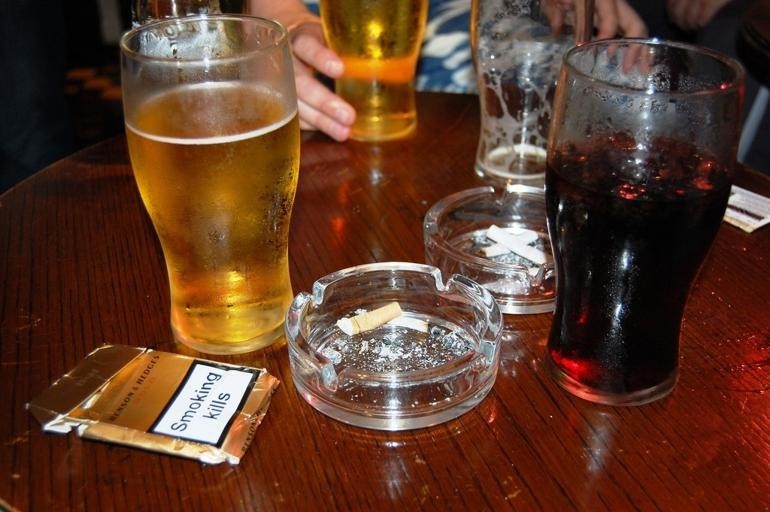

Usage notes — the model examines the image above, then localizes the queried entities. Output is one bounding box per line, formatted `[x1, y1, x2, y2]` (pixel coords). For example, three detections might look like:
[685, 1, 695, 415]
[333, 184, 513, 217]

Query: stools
[724, 20, 769, 168]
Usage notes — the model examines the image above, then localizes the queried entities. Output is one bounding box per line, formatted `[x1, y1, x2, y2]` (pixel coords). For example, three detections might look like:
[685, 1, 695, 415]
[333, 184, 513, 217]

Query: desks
[1, 88, 769, 512]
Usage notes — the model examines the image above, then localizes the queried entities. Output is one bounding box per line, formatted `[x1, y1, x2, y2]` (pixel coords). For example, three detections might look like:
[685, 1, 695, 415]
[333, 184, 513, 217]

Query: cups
[545, 37, 745, 407]
[467, 2, 576, 194]
[118, 13, 301, 355]
[315, 0, 430, 144]
